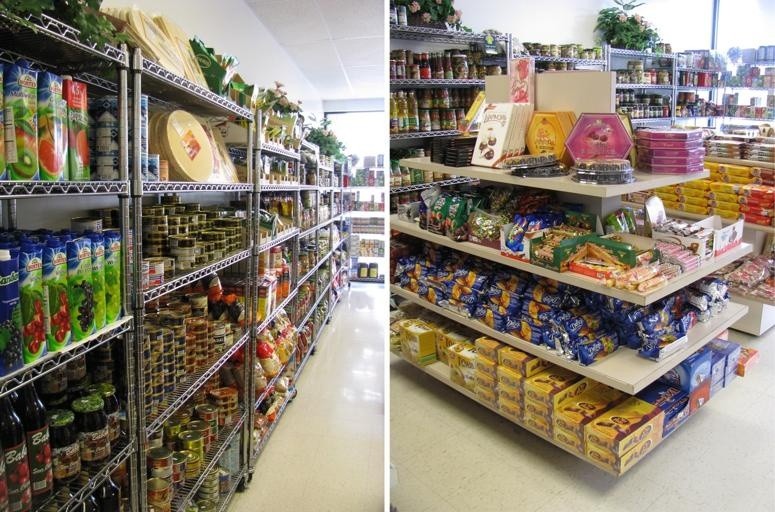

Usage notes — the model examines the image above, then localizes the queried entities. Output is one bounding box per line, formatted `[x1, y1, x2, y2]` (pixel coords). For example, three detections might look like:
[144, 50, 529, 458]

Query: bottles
[390, 49, 454, 79]
[390, 148, 443, 187]
[387, 88, 419, 133]
[390, 0, 408, 26]
[0, 375, 123, 512]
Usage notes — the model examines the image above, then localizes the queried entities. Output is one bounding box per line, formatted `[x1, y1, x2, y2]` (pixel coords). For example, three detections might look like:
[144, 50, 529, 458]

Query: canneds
[177, 370, 239, 463]
[198, 465, 230, 505]
[84, 383, 122, 445]
[100, 195, 243, 290]
[146, 418, 201, 509]
[142, 293, 240, 414]
[72, 395, 111, 465]
[48, 409, 82, 484]
